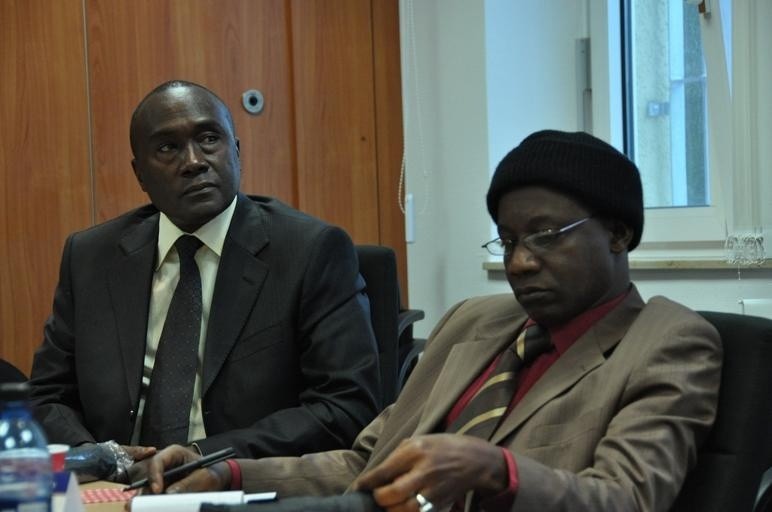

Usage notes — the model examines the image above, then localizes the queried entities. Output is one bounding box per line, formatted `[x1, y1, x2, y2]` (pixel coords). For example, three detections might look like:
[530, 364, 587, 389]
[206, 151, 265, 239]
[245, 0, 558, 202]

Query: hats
[486, 129, 644, 251]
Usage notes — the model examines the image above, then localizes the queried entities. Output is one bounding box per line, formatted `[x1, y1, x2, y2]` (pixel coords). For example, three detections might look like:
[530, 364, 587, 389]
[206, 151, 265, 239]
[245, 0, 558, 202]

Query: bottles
[0, 384, 54, 511]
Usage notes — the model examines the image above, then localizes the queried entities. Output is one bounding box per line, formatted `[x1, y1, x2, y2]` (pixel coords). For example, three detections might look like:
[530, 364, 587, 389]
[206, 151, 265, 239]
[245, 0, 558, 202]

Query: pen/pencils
[123, 446, 236, 491]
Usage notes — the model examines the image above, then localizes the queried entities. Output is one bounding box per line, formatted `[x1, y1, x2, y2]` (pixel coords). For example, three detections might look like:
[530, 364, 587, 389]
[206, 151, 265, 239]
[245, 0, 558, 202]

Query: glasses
[480, 216, 595, 258]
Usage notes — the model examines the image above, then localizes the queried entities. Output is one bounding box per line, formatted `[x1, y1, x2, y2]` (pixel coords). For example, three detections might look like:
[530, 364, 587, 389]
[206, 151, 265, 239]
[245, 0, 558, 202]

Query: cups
[46, 444, 70, 473]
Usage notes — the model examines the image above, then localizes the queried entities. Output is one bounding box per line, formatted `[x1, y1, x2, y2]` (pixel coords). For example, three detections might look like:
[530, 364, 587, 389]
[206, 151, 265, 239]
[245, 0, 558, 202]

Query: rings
[414, 493, 433, 511]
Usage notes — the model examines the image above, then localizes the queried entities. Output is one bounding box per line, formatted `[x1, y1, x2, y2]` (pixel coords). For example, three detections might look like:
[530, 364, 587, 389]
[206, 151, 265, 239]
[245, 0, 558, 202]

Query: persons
[28, 79, 381, 485]
[122, 130, 724, 512]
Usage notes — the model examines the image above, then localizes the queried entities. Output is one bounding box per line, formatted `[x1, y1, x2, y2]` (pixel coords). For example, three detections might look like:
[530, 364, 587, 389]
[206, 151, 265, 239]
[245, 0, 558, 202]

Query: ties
[444, 321, 551, 442]
[136, 233, 204, 451]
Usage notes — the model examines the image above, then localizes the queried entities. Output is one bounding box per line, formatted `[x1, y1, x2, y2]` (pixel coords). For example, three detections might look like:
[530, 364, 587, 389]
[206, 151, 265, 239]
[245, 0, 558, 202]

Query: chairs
[668, 311, 771, 512]
[353, 245, 426, 408]
